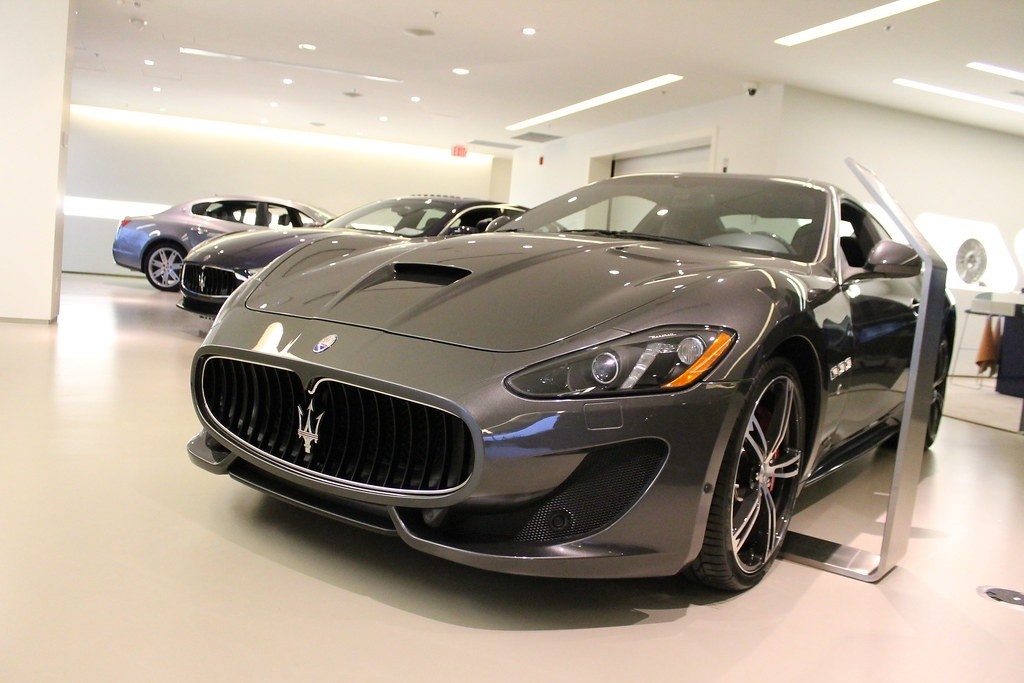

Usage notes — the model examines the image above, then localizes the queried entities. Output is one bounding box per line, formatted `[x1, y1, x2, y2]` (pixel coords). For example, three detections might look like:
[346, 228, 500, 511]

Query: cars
[177, 195, 568, 319]
[187, 172, 957, 595]
[113, 193, 355, 294]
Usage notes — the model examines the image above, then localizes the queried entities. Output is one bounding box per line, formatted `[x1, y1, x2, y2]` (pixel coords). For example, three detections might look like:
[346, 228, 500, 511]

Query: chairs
[630, 191, 725, 241]
[791, 225, 844, 261]
